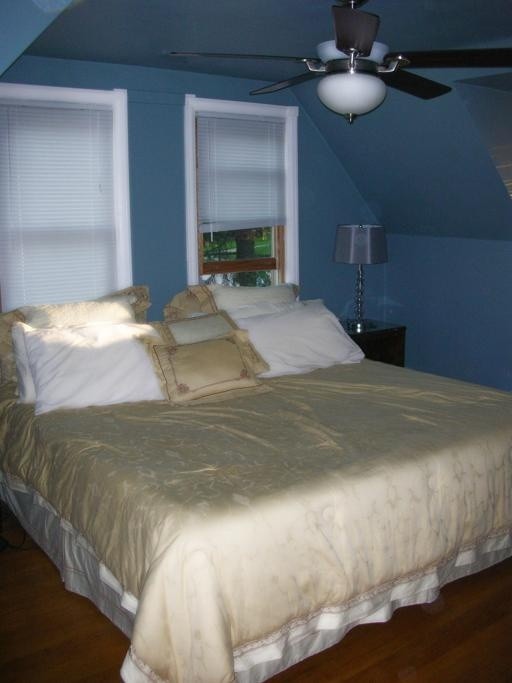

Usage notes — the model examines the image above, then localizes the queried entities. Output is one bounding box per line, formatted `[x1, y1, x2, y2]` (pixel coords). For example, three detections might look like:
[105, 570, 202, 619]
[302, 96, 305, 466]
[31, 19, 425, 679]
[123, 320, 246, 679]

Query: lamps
[332, 223, 389, 328]
[317, 74, 386, 124]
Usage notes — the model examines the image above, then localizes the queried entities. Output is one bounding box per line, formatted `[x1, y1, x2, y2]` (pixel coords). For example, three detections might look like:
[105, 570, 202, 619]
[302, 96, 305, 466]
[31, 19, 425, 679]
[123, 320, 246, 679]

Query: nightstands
[340, 318, 407, 366]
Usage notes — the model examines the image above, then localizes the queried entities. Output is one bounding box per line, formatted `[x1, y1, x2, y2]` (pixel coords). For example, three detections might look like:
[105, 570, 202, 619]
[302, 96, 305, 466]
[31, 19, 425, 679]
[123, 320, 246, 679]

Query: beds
[0, 355, 512, 682]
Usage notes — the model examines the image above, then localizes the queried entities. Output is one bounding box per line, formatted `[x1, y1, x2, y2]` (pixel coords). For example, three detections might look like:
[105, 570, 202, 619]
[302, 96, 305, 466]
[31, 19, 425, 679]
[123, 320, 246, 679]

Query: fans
[166, 0, 512, 100]
